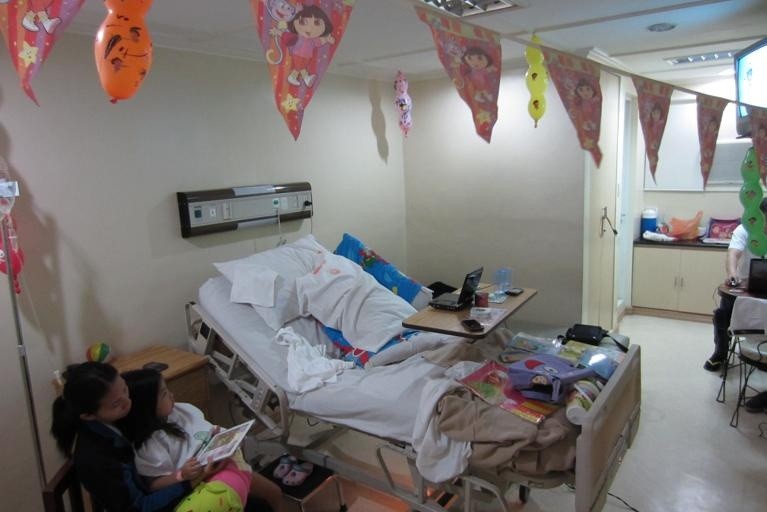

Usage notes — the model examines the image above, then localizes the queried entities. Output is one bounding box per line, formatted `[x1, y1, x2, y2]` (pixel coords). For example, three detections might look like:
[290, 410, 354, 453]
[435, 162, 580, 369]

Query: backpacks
[507, 353, 593, 402]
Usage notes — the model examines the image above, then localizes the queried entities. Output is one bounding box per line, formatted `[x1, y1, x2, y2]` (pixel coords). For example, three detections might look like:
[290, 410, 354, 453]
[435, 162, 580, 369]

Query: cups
[475, 293, 489, 307]
[493, 268, 511, 297]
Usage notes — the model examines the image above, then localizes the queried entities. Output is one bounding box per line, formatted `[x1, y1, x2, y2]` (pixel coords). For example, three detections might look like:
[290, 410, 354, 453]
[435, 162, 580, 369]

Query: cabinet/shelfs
[55, 345, 213, 427]
[630, 244, 738, 318]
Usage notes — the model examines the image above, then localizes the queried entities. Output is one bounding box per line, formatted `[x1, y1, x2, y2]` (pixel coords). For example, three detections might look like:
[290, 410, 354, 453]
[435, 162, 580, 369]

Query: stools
[255, 451, 345, 511]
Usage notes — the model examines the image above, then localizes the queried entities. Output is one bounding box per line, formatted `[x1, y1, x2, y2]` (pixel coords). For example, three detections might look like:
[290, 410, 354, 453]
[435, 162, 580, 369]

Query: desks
[402, 281, 537, 369]
[715, 277, 766, 305]
[729, 340, 766, 437]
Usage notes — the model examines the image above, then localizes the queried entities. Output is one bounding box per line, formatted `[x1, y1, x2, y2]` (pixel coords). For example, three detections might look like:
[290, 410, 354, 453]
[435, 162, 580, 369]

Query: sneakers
[745, 389, 767, 415]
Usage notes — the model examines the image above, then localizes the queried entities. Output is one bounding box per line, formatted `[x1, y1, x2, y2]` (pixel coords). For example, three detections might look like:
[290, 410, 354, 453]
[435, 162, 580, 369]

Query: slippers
[702, 357, 723, 371]
[281, 461, 314, 486]
[272, 455, 297, 480]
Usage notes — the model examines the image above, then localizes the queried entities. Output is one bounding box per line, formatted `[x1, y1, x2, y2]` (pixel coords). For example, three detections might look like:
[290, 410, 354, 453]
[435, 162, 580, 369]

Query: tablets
[195, 417, 255, 467]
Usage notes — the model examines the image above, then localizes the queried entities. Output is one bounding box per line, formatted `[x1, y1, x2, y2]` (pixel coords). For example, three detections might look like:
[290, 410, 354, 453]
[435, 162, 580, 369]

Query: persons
[705, 197, 767, 372]
[50, 362, 223, 512]
[119, 370, 288, 511]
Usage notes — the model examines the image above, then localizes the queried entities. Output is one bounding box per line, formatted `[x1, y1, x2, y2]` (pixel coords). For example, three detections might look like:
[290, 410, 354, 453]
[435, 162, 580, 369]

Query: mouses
[729, 278, 740, 287]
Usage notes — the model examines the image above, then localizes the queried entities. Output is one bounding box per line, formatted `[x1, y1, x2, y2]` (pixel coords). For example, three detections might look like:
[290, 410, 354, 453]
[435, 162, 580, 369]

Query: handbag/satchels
[566, 323, 605, 346]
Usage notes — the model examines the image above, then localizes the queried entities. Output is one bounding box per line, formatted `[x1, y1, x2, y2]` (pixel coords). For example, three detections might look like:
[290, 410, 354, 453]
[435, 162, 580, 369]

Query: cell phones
[462, 319, 484, 332]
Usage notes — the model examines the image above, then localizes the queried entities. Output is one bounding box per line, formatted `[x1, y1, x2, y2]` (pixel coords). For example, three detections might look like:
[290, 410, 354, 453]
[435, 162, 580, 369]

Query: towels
[275, 325, 356, 395]
[228, 268, 277, 307]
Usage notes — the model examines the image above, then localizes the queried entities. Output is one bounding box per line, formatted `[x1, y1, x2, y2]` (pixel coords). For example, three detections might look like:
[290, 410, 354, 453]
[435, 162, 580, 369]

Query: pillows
[295, 255, 418, 354]
[212, 233, 362, 331]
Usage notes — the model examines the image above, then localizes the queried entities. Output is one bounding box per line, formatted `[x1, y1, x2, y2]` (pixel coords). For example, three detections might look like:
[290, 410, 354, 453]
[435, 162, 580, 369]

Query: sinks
[702, 236, 732, 244]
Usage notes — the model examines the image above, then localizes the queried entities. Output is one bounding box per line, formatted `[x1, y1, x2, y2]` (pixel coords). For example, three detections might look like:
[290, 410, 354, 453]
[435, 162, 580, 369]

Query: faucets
[725, 226, 733, 236]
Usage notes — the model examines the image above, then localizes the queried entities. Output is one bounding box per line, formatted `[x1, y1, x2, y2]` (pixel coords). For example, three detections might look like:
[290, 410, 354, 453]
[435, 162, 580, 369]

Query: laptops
[747, 258, 767, 294]
[428, 266, 483, 312]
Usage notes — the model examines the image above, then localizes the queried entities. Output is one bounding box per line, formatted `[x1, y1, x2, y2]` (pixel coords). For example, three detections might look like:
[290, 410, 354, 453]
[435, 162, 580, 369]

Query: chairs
[714, 295, 766, 427]
[42, 454, 94, 511]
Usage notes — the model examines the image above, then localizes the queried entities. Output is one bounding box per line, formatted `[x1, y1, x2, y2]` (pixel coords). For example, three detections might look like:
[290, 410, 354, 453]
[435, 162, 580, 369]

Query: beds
[182, 234, 643, 510]
